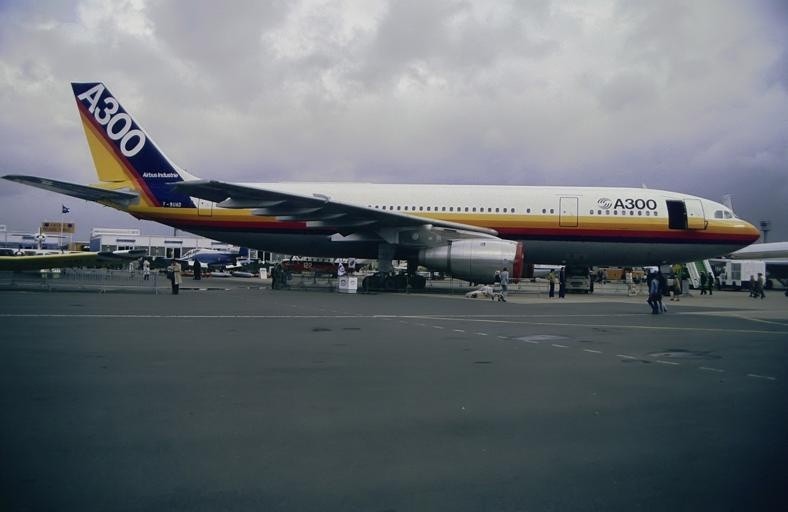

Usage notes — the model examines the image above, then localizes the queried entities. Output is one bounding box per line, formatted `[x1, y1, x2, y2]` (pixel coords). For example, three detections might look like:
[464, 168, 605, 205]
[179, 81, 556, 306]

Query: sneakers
[652, 310, 668, 314]
[492, 297, 506, 302]
[749, 295, 766, 299]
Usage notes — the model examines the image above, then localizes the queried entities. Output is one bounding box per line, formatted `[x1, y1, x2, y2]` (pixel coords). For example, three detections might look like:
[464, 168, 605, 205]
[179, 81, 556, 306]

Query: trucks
[562, 263, 593, 294]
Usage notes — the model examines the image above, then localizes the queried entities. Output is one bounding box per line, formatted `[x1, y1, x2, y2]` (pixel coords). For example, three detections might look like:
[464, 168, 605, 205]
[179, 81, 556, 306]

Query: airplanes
[1, 81, 762, 291]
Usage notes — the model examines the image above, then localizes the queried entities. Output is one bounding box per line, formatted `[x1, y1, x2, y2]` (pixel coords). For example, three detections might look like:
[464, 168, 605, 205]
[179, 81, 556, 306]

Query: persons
[597, 268, 609, 287]
[625, 268, 637, 296]
[193, 258, 201, 280]
[129, 256, 151, 280]
[547, 269, 555, 297]
[559, 267, 565, 298]
[647, 271, 668, 314]
[167, 259, 182, 294]
[670, 272, 682, 301]
[700, 271, 713, 295]
[748, 273, 766, 299]
[271, 268, 280, 290]
[494, 267, 509, 301]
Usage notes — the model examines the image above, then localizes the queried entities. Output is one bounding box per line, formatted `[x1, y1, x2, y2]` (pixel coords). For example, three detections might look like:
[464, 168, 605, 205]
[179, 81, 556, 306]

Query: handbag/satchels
[164, 272, 174, 279]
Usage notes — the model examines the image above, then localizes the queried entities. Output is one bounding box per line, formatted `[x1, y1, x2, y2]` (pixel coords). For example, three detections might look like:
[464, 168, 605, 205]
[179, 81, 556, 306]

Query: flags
[335, 260, 346, 277]
[62, 205, 71, 213]
